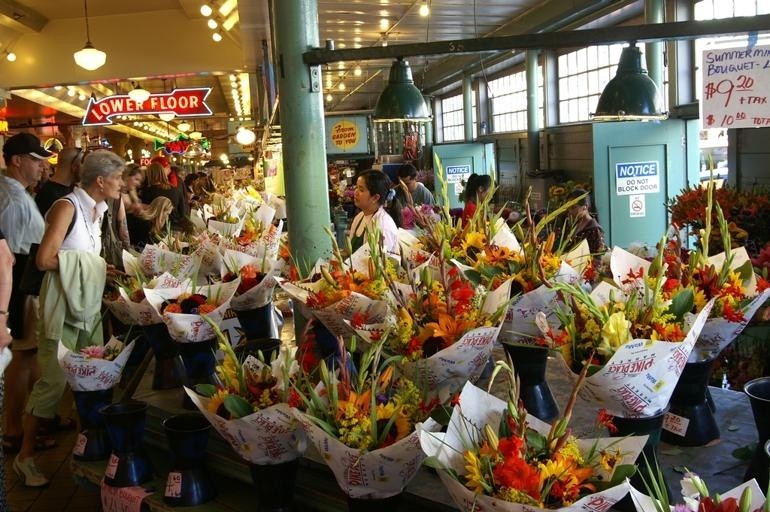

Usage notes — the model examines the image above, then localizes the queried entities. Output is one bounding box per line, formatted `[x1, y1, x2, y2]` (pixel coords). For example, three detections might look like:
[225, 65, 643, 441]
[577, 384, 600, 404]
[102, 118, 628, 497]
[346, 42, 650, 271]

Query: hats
[2, 132, 55, 160]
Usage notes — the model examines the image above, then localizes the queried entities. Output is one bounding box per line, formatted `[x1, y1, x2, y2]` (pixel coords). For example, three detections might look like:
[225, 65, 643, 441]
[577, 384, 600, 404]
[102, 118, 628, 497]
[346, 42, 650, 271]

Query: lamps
[212, 30, 225, 43]
[129, 84, 150, 104]
[371, 57, 434, 124]
[591, 43, 669, 121]
[189, 121, 202, 138]
[3, 48, 17, 64]
[199, 0, 215, 17]
[176, 120, 190, 132]
[73, 0, 110, 71]
[159, 112, 177, 122]
[206, 15, 221, 31]
[229, 74, 246, 116]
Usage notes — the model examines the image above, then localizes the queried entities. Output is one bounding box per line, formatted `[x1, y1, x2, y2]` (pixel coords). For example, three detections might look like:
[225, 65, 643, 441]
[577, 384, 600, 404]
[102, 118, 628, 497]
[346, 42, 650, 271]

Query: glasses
[72, 147, 83, 163]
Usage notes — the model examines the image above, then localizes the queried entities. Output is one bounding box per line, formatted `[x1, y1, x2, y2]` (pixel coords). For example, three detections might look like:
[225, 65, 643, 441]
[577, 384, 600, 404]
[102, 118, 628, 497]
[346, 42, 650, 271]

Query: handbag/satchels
[101, 200, 123, 267]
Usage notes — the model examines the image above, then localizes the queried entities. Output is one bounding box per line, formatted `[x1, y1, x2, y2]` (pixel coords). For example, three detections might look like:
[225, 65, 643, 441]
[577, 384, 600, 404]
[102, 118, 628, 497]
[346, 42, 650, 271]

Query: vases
[745, 378, 770, 451]
[236, 302, 286, 338]
[502, 343, 559, 424]
[610, 407, 667, 496]
[73, 394, 112, 461]
[175, 344, 228, 410]
[146, 324, 182, 391]
[160, 410, 213, 508]
[100, 402, 148, 486]
[660, 361, 721, 448]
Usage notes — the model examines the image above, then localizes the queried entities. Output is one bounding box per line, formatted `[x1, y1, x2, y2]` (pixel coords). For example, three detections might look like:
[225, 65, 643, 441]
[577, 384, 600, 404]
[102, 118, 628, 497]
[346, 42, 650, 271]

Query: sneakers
[12, 453, 50, 487]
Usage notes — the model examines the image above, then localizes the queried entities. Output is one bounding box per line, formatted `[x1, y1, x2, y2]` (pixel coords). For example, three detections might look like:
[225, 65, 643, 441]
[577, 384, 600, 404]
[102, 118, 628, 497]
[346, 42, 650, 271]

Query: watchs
[1, 311, 9, 318]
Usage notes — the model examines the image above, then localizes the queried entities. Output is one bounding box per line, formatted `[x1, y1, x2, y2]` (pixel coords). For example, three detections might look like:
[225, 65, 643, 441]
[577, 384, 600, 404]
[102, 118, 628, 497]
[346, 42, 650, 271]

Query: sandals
[35, 410, 76, 433]
[2, 433, 55, 457]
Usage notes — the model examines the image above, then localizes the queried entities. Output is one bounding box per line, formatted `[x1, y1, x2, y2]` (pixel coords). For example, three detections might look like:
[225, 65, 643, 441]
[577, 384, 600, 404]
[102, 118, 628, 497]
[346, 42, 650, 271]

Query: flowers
[611, 156, 769, 363]
[122, 241, 204, 282]
[290, 323, 444, 498]
[416, 360, 650, 512]
[222, 249, 287, 309]
[142, 274, 239, 342]
[451, 183, 594, 341]
[57, 326, 140, 393]
[345, 177, 516, 404]
[118, 271, 192, 325]
[403, 152, 518, 248]
[628, 451, 769, 511]
[275, 224, 361, 345]
[537, 275, 718, 416]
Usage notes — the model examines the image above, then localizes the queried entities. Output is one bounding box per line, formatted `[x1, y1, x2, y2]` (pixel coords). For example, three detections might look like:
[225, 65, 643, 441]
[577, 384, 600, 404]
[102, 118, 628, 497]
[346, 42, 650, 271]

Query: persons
[34, 147, 82, 216]
[392, 164, 435, 207]
[185, 173, 199, 200]
[0, 132, 77, 453]
[198, 172, 208, 194]
[152, 157, 187, 196]
[563, 190, 605, 255]
[0, 229, 14, 353]
[14, 149, 130, 486]
[463, 174, 497, 230]
[141, 163, 186, 220]
[124, 197, 172, 250]
[350, 169, 397, 255]
[106, 162, 144, 254]
